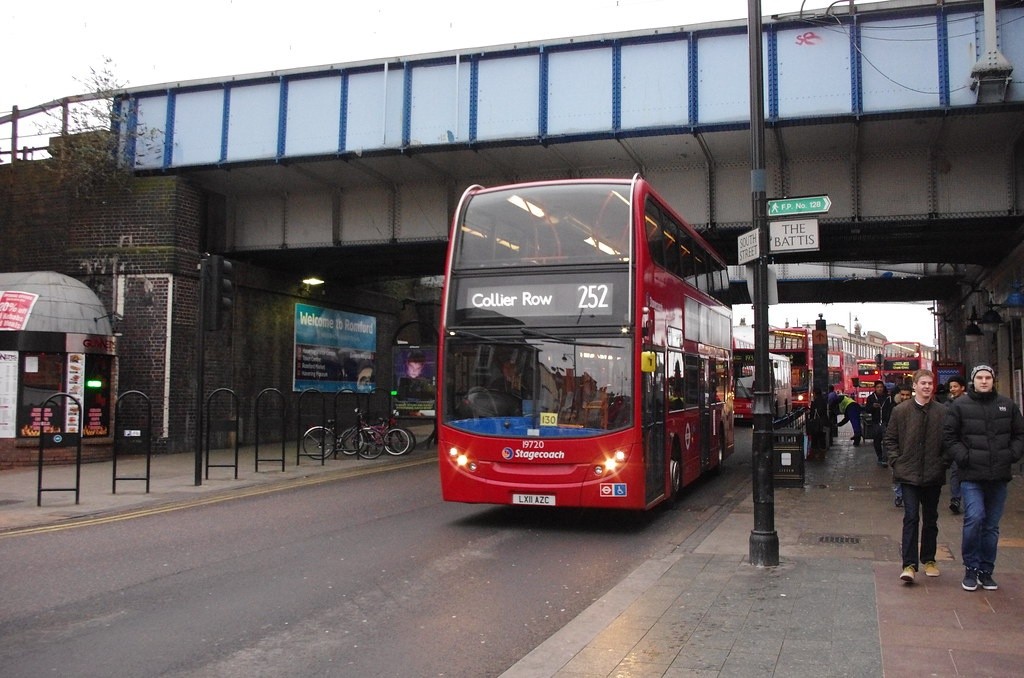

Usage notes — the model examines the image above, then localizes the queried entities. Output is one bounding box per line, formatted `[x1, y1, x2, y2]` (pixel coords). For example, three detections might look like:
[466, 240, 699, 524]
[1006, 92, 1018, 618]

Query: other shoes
[877, 457, 884, 465]
[881, 459, 887, 467]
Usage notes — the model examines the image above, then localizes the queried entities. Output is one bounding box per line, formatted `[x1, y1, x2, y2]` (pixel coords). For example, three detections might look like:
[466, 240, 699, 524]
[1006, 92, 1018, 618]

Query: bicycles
[301, 409, 416, 460]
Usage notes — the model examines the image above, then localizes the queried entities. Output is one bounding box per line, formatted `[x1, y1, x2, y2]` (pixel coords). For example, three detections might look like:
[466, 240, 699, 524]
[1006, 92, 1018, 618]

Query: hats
[970, 364, 996, 381]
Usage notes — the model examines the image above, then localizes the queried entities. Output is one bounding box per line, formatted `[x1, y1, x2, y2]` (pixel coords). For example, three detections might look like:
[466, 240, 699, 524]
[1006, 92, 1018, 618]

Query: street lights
[562, 353, 577, 378]
[621, 376, 627, 398]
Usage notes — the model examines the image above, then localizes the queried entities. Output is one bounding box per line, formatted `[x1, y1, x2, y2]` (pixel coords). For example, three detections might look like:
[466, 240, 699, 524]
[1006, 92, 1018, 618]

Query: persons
[943, 376, 968, 512]
[407, 351, 426, 379]
[885, 385, 912, 506]
[490, 358, 528, 399]
[883, 370, 954, 581]
[830, 395, 861, 446]
[865, 381, 890, 462]
[827, 385, 838, 437]
[934, 384, 946, 404]
[807, 387, 826, 461]
[942, 365, 1024, 590]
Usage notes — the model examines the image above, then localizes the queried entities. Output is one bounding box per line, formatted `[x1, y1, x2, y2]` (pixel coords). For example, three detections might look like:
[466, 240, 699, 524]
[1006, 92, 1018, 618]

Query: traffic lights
[205, 254, 233, 331]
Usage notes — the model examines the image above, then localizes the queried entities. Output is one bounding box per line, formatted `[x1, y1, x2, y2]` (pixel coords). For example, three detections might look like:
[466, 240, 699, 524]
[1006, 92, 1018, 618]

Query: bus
[733, 327, 937, 425]
[437, 172, 736, 511]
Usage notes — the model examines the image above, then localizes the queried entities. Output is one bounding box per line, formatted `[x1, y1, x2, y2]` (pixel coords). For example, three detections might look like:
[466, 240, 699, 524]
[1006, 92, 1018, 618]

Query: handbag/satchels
[806, 408, 824, 435]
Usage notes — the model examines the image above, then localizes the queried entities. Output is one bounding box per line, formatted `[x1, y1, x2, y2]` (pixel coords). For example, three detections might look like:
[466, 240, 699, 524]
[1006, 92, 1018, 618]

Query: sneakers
[977, 571, 998, 590]
[923, 561, 941, 577]
[948, 497, 961, 513]
[899, 564, 916, 581]
[962, 567, 978, 590]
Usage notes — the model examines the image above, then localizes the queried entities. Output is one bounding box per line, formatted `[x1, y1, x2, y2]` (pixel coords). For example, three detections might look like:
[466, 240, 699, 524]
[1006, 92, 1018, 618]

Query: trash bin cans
[773, 429, 805, 489]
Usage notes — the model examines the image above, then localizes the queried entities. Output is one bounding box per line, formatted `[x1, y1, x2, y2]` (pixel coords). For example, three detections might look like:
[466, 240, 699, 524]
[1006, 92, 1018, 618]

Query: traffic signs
[766, 194, 831, 217]
